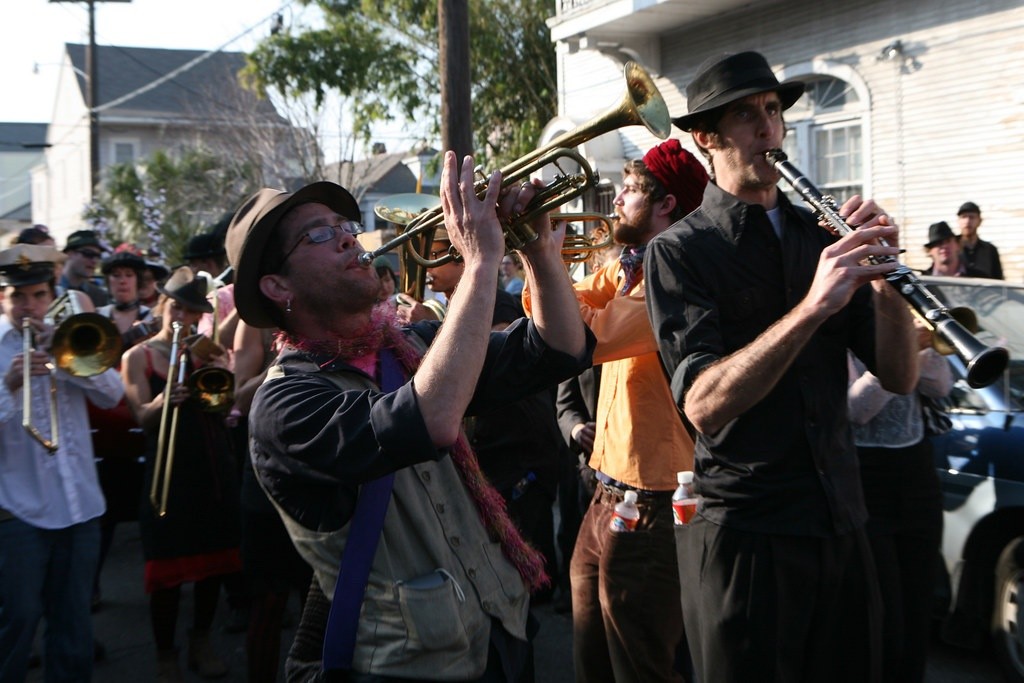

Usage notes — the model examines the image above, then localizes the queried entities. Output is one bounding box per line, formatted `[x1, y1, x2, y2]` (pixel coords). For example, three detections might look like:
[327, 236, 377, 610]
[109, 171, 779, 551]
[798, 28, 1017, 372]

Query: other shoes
[156, 644, 182, 683]
[280, 590, 301, 627]
[186, 626, 228, 679]
[222, 604, 246, 632]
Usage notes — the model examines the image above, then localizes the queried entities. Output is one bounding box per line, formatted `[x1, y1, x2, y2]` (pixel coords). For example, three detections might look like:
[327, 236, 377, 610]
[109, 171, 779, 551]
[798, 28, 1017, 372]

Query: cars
[858, 277, 1024, 682]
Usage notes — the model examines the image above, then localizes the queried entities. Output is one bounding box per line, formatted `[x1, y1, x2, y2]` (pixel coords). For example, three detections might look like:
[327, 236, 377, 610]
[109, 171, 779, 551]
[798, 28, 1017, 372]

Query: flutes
[760, 146, 1013, 390]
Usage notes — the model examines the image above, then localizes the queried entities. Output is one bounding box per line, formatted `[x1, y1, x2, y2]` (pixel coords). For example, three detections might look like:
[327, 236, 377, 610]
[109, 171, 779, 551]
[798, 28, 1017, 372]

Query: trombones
[150, 323, 233, 523]
[22, 288, 122, 452]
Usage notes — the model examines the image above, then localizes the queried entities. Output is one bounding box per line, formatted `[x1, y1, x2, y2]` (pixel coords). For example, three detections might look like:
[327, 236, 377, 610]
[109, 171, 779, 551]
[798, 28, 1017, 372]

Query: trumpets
[356, 62, 670, 277]
[546, 213, 622, 265]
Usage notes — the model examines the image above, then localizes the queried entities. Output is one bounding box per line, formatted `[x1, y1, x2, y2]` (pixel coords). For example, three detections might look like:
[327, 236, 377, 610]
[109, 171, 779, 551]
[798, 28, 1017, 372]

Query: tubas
[373, 192, 439, 304]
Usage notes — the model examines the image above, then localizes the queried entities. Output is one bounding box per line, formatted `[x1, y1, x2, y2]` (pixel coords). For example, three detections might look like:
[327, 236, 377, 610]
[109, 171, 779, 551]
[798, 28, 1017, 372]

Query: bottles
[609, 490, 640, 532]
[672, 471, 704, 526]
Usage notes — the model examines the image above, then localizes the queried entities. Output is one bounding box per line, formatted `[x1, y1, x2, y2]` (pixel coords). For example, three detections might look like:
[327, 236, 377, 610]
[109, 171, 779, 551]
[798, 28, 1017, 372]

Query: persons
[0, 213, 955, 683]
[222, 153, 595, 683]
[521, 140, 709, 683]
[644, 49, 920, 683]
[925, 222, 971, 277]
[955, 203, 1002, 280]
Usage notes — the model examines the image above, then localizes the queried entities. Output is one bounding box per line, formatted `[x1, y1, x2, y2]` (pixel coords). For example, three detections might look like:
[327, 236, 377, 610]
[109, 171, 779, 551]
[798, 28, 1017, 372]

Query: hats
[642, 138, 710, 212]
[957, 202, 980, 215]
[101, 251, 146, 274]
[155, 266, 215, 313]
[62, 230, 112, 254]
[1, 246, 55, 287]
[924, 221, 961, 249]
[670, 49, 805, 133]
[225, 181, 361, 329]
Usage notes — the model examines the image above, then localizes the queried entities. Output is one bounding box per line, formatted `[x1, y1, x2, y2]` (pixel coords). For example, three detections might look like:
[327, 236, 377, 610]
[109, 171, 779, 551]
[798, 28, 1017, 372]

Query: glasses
[69, 248, 101, 260]
[429, 247, 448, 260]
[279, 221, 364, 263]
